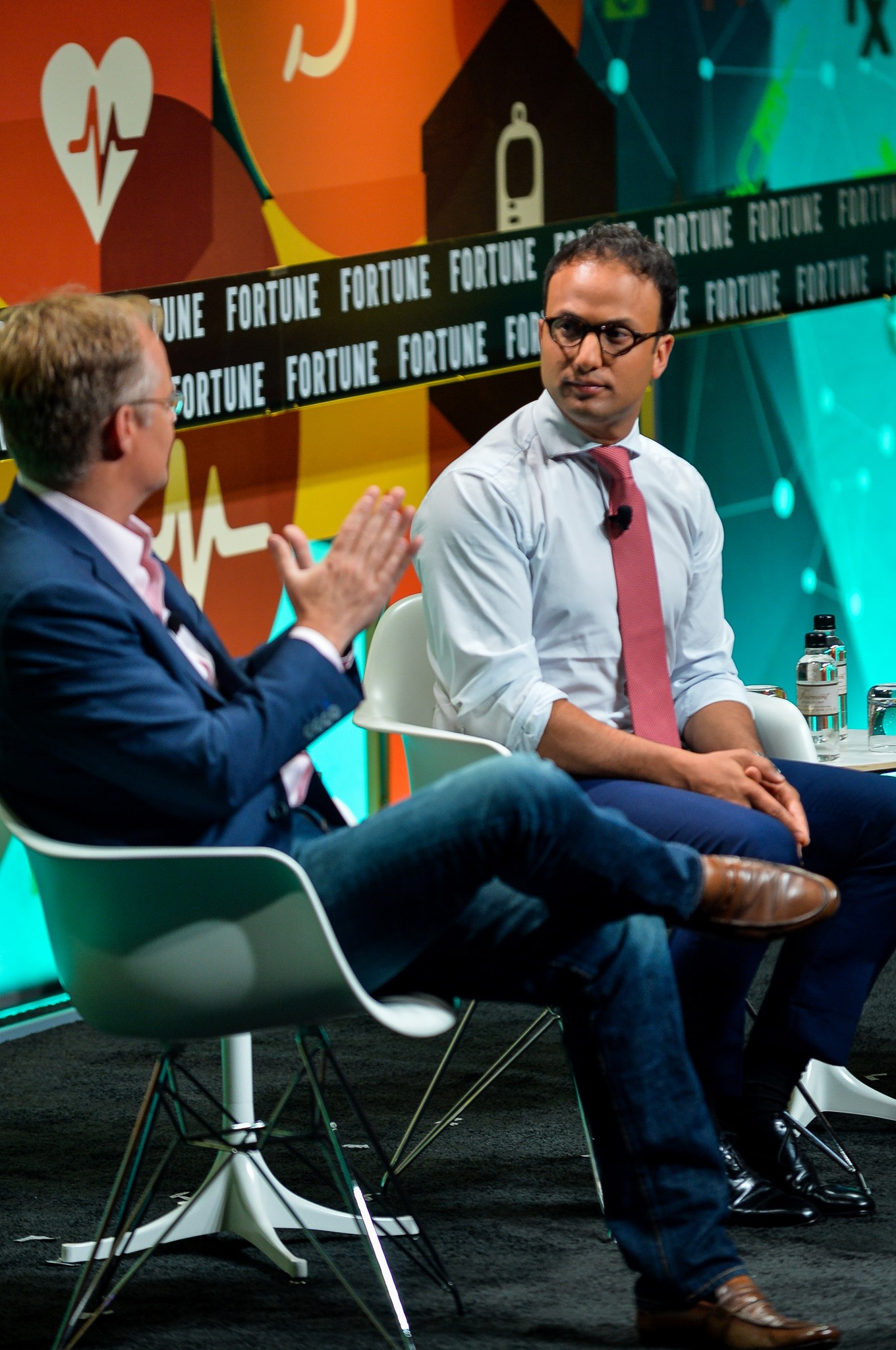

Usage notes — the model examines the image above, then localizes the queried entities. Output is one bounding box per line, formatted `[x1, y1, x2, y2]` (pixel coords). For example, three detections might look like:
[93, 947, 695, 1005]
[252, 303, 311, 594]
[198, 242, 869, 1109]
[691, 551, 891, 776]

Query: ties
[592, 443, 681, 757]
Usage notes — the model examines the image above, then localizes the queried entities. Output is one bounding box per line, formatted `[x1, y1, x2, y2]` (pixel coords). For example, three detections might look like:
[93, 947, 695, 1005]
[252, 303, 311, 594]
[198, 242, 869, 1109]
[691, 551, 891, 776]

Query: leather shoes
[716, 1144, 823, 1226]
[728, 1117, 874, 1216]
[695, 855, 841, 936]
[634, 1271, 838, 1350]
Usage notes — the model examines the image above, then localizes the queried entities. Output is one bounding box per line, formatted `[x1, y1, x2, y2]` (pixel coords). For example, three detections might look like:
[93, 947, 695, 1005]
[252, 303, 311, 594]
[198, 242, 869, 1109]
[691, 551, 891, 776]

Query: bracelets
[754, 751, 781, 774]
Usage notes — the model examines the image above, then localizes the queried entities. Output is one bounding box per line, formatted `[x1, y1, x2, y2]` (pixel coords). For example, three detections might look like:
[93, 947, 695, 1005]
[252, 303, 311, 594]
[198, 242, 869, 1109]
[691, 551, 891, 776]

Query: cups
[867, 684, 896, 754]
[744, 684, 788, 700]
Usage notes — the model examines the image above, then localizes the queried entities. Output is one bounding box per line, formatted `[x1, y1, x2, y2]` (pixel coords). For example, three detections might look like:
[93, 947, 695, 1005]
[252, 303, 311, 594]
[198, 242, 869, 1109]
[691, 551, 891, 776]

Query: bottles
[812, 614, 848, 741]
[796, 632, 841, 762]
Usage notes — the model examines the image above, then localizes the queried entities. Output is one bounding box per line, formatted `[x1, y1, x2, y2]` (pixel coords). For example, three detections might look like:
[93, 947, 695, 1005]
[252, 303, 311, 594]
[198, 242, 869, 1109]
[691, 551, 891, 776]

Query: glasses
[115, 392, 186, 418]
[541, 315, 666, 358]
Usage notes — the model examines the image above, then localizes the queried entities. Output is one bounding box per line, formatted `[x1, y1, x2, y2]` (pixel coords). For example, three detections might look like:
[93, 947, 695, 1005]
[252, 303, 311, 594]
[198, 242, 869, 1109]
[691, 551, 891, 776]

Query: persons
[410, 223, 895, 1222]
[0, 289, 838, 1349]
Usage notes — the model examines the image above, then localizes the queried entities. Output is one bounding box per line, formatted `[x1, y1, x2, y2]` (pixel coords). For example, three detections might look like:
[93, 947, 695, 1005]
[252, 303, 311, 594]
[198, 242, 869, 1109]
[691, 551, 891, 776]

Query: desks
[787, 728, 896, 1137]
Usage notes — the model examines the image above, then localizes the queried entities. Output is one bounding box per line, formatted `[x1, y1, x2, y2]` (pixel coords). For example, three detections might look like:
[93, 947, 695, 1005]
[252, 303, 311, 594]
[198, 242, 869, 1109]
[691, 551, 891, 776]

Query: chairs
[352, 591, 754, 1243]
[0, 792, 459, 1284]
